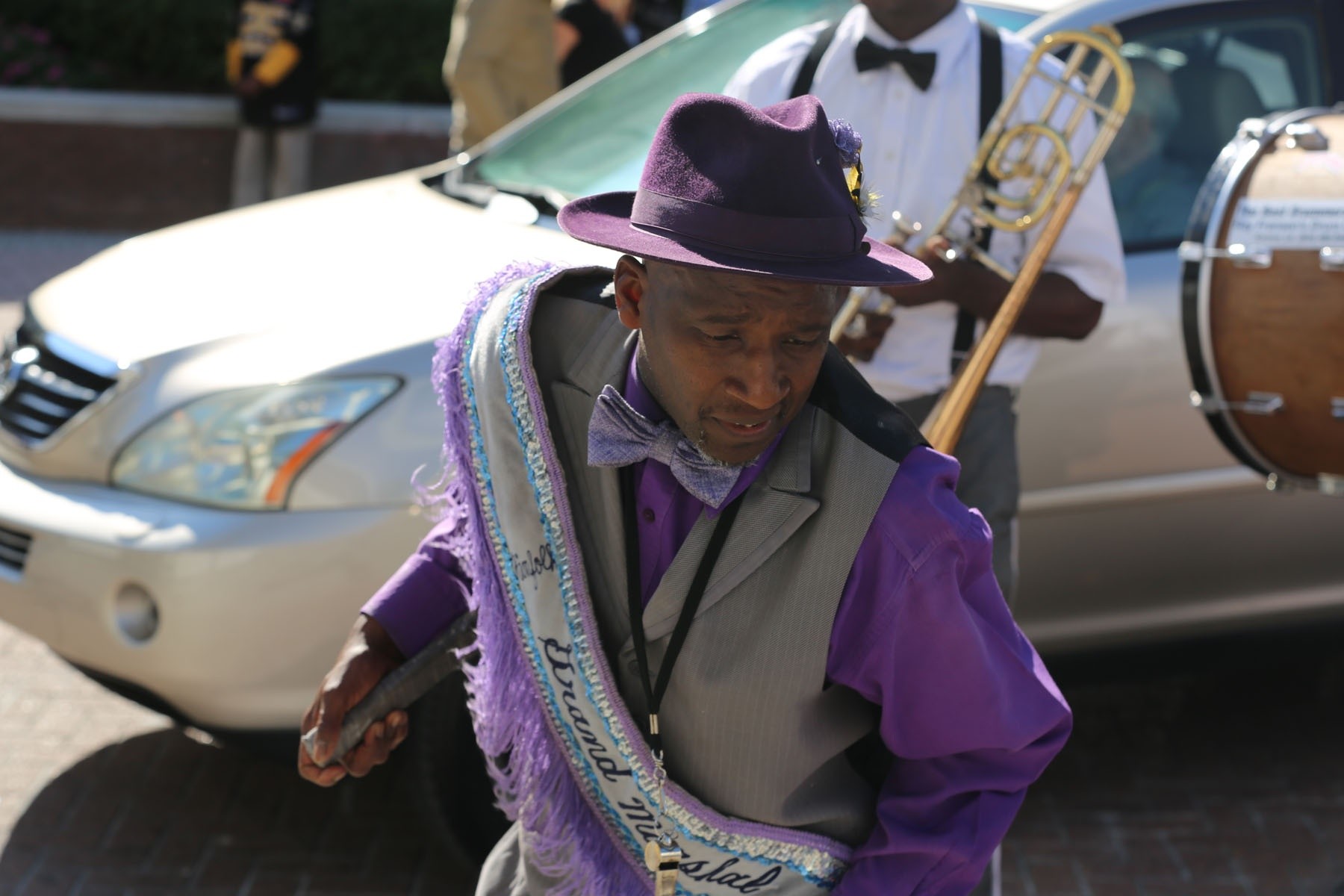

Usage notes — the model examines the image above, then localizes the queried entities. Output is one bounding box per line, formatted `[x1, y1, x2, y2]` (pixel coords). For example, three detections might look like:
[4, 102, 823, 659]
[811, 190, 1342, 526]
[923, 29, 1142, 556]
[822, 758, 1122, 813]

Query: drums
[1179, 102, 1344, 486]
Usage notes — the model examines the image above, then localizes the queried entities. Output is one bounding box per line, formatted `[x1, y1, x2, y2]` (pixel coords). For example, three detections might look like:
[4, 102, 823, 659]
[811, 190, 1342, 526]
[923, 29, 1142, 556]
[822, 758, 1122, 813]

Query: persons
[551, 0, 721, 92]
[226, 0, 315, 211]
[298, 93, 1073, 896]
[723, 0, 1128, 896]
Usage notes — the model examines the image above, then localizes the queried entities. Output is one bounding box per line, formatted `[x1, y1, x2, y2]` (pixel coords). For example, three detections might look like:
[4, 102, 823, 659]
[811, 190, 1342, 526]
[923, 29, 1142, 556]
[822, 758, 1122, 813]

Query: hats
[557, 91, 934, 287]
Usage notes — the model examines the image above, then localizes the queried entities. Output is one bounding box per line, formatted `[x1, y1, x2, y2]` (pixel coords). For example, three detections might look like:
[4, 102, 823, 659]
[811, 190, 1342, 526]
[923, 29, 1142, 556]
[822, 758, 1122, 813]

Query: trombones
[829, 23, 1137, 458]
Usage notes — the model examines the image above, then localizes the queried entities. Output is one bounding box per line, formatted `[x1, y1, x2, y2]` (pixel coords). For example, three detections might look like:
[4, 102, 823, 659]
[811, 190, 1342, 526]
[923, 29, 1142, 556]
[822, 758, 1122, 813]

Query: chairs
[1163, 57, 1263, 177]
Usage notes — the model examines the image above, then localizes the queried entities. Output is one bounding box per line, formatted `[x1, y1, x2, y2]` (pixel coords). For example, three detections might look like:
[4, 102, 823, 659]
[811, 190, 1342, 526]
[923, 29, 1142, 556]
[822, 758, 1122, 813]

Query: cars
[1, 1, 1344, 836]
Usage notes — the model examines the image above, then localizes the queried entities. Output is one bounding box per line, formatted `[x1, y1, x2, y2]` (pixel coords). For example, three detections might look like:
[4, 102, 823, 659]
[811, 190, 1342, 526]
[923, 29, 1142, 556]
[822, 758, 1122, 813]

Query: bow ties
[586, 384, 761, 509]
[856, 36, 937, 94]
